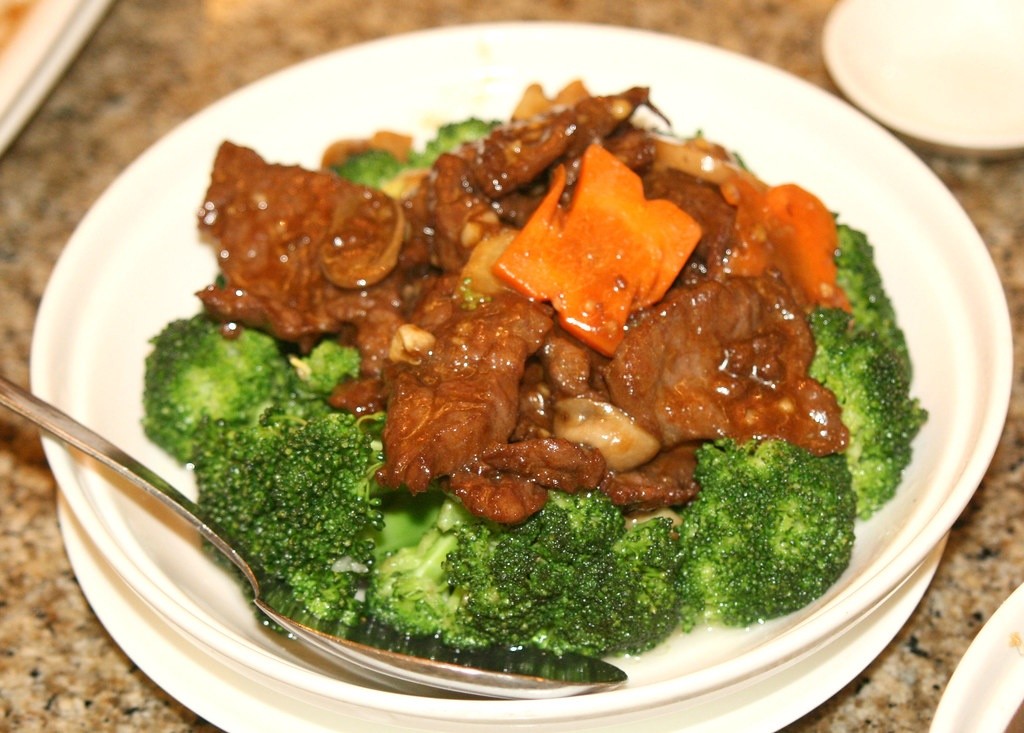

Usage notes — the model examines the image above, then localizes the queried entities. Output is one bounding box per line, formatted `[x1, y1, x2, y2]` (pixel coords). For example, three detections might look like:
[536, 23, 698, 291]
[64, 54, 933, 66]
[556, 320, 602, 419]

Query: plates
[822, 0, 1024, 157]
[0, 0, 118, 160]
[928, 580, 1024, 733]
[46, 482, 950, 733]
[30, 22, 1012, 722]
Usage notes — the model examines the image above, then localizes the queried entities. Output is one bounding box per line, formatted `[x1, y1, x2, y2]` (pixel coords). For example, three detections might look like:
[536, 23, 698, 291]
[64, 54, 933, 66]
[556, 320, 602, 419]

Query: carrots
[493, 161, 662, 359]
[722, 176, 853, 315]
[564, 144, 703, 314]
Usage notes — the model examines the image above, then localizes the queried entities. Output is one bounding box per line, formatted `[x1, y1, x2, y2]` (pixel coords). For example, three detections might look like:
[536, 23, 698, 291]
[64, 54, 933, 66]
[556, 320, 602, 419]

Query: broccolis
[142, 117, 932, 660]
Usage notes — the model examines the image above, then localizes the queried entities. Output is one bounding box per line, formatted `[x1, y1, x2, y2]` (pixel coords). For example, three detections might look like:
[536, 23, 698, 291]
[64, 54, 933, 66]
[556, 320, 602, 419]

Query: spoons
[0, 375, 629, 702]
